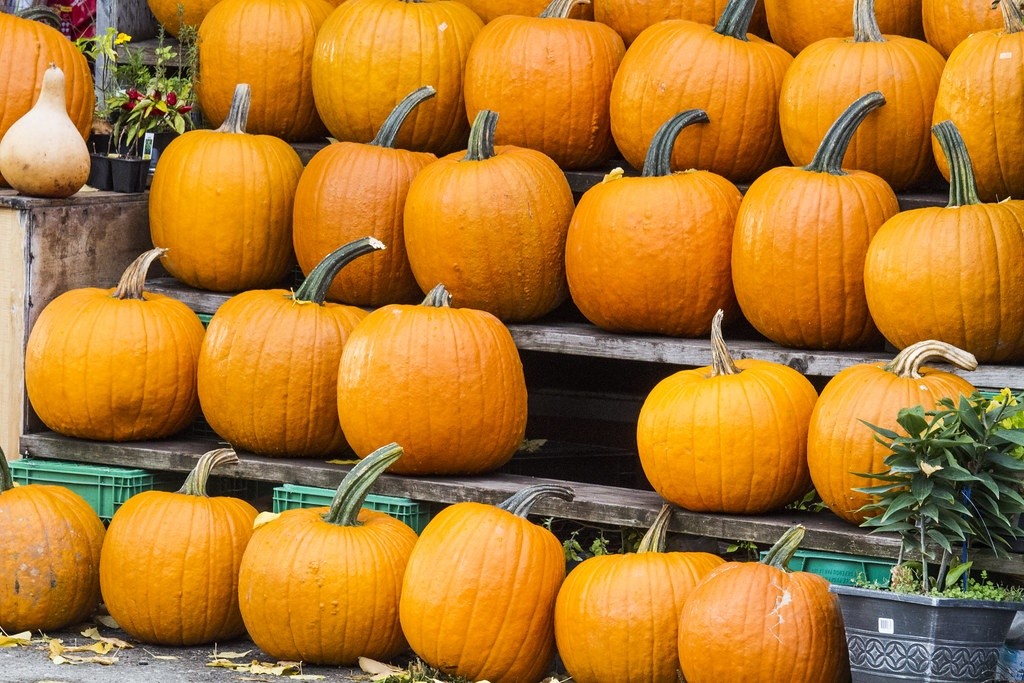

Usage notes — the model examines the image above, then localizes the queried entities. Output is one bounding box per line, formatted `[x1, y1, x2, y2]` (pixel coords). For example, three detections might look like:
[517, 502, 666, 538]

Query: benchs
[22, 141, 1024, 575]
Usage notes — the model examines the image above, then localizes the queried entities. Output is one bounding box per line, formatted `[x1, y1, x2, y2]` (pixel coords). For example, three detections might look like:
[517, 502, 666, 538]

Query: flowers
[103, 87, 192, 160]
[861, 386, 1023, 600]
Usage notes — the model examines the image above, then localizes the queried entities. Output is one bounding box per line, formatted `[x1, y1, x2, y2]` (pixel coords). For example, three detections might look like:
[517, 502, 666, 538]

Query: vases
[827, 586, 1024, 683]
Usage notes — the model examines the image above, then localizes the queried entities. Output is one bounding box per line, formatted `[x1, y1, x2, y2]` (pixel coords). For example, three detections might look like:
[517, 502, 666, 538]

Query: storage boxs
[7, 457, 155, 522]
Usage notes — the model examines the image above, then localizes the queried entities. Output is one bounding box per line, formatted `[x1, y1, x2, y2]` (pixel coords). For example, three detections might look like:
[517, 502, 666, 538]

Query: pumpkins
[0, 0, 1021, 682]
